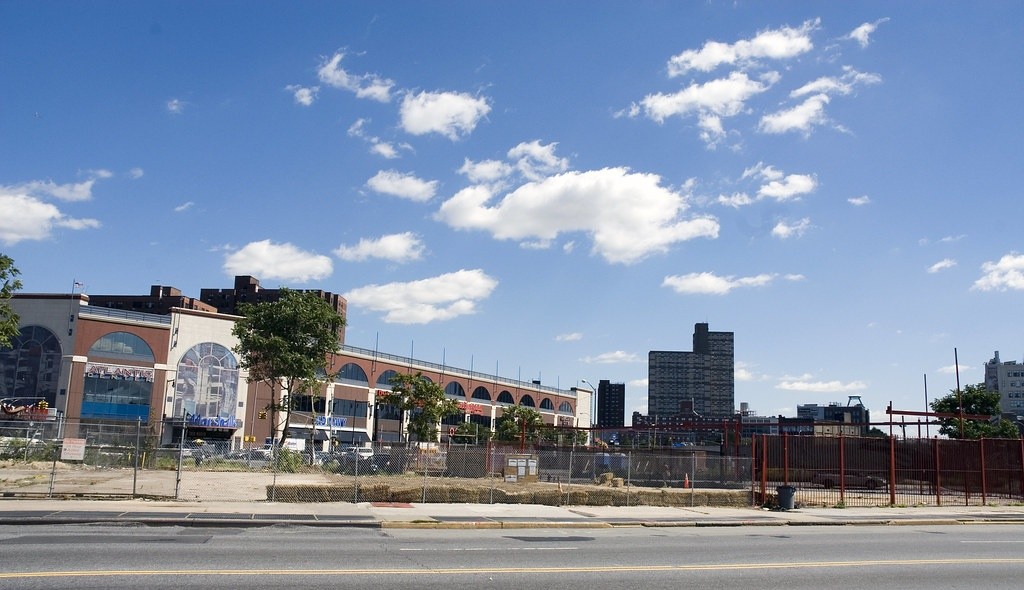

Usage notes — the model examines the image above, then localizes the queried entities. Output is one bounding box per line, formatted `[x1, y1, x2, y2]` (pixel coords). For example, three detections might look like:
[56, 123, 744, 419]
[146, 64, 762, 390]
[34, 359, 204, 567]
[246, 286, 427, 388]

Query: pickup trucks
[255, 444, 278, 461]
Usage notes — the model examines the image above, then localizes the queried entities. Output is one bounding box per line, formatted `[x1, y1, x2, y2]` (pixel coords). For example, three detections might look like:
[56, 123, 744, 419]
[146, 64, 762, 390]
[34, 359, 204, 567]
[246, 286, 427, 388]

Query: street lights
[582, 378, 597, 427]
[373, 399, 380, 441]
[1013, 421, 1023, 426]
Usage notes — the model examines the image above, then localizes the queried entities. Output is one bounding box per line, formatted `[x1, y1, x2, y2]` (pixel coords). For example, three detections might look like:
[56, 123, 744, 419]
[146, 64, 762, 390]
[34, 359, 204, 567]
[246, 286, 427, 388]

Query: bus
[346, 447, 375, 461]
[85, 445, 136, 460]
[0, 397, 46, 409]
[192, 439, 206, 447]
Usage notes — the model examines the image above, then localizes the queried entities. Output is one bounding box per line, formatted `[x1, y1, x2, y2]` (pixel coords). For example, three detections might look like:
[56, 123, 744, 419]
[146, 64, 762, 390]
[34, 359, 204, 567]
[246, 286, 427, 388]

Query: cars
[429, 452, 447, 467]
[817, 470, 887, 490]
[225, 444, 257, 460]
[314, 451, 345, 462]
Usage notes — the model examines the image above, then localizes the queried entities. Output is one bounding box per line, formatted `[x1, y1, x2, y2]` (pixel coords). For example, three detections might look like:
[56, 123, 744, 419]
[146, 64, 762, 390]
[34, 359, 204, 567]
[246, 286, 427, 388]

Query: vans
[0, 436, 48, 454]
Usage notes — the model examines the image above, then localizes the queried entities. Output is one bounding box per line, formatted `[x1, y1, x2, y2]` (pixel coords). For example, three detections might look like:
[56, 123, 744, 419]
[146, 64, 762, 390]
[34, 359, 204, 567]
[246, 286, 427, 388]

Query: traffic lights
[333, 441, 338, 446]
[258, 411, 262, 419]
[263, 412, 267, 420]
[38, 401, 49, 410]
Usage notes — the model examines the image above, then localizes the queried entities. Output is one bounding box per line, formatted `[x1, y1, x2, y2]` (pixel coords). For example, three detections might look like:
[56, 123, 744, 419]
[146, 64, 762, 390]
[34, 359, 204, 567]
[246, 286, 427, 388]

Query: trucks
[265, 437, 305, 454]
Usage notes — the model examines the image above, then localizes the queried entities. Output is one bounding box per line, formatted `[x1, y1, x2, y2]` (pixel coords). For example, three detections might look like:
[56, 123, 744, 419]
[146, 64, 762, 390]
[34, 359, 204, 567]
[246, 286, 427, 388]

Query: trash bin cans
[777, 485, 796, 509]
[670, 480, 679, 488]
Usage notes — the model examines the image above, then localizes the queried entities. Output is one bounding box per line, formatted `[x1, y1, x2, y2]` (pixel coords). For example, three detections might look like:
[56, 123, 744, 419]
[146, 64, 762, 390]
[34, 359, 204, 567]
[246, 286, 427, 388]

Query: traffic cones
[683, 472, 689, 488]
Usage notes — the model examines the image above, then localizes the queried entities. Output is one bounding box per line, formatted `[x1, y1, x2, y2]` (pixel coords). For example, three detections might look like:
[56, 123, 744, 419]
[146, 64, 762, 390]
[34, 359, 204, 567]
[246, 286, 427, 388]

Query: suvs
[157, 444, 192, 458]
